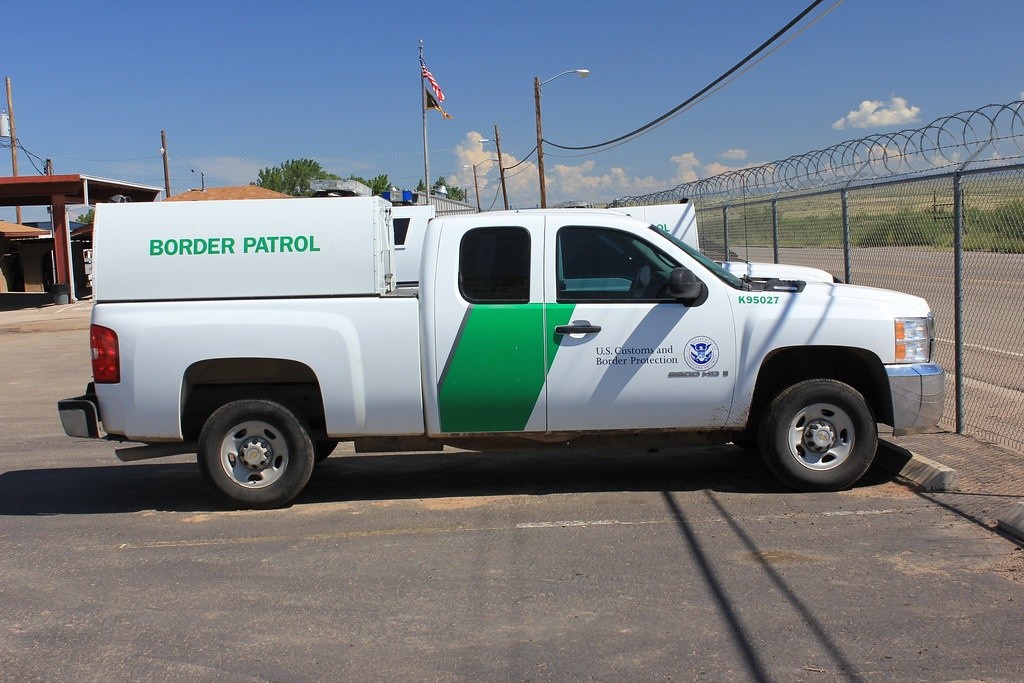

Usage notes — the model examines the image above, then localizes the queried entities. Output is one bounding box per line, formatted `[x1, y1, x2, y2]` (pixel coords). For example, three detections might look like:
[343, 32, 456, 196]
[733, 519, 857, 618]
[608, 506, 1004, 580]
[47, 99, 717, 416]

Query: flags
[425, 86, 454, 119]
[419, 55, 446, 103]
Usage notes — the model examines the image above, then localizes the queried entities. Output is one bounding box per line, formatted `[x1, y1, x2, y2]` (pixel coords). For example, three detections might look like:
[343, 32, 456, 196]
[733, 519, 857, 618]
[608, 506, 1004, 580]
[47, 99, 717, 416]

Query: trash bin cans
[51, 284, 69, 305]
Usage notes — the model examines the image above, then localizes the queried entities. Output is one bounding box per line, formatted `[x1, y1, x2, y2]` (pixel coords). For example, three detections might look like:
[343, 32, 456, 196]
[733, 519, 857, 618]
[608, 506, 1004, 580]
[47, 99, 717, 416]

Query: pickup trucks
[386, 200, 842, 320]
[57, 188, 946, 493]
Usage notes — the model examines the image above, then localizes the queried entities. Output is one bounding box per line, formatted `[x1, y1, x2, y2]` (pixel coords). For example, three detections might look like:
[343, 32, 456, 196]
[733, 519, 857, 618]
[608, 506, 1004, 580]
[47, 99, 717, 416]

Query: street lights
[478, 137, 513, 210]
[534, 67, 589, 208]
[473, 157, 499, 212]
